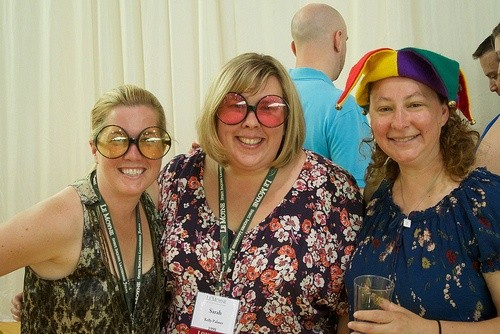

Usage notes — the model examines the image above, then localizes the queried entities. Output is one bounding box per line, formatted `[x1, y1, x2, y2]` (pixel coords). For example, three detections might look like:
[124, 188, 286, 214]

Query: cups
[353, 275, 395, 333]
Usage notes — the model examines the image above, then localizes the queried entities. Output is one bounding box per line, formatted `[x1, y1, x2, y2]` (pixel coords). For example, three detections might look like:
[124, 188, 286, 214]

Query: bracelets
[435, 318, 444, 334]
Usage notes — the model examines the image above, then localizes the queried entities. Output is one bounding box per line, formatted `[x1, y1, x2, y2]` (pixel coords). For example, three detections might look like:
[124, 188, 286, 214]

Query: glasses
[92, 124, 171, 161]
[217, 92, 291, 129]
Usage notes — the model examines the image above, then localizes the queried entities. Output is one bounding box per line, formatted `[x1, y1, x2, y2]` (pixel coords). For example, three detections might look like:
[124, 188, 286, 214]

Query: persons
[0, 84, 170, 334]
[473, 22, 500, 96]
[9, 52, 365, 334]
[287, 3, 372, 193]
[338, 47, 500, 334]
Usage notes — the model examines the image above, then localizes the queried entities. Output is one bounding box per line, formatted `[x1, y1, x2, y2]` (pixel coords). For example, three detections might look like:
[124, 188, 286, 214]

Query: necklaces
[400, 162, 448, 228]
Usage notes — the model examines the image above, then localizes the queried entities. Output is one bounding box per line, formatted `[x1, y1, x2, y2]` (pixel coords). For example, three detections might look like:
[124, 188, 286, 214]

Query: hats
[336, 46, 476, 127]
